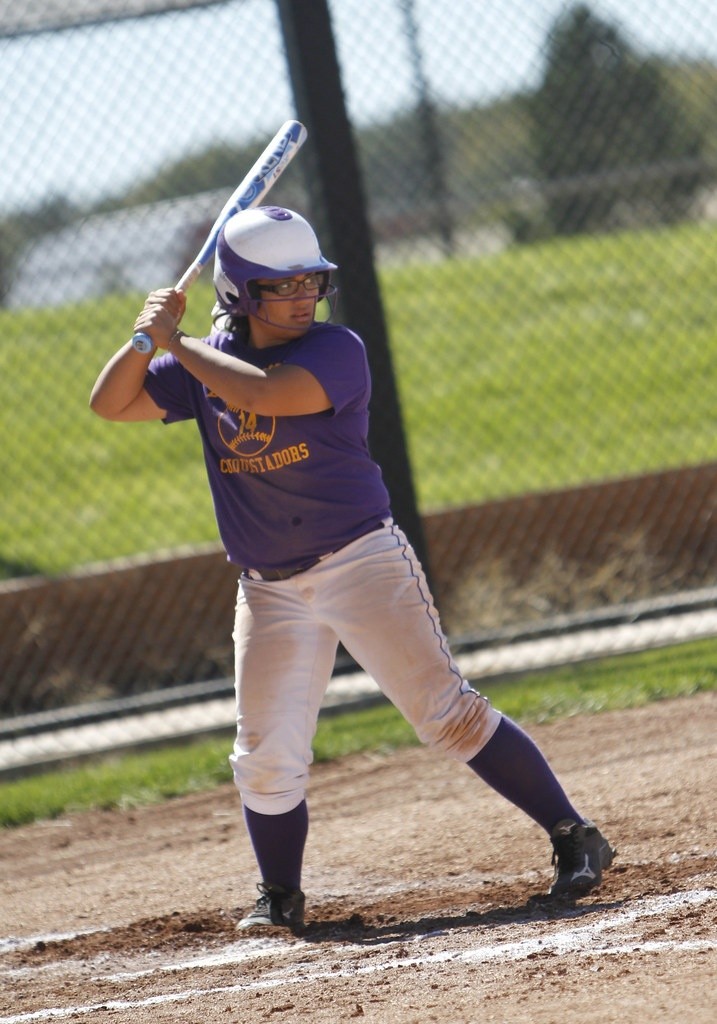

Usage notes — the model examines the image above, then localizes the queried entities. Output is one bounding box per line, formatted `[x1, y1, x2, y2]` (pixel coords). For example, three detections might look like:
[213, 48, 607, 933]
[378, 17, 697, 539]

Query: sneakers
[548, 818, 618, 896]
[235, 882, 305, 931]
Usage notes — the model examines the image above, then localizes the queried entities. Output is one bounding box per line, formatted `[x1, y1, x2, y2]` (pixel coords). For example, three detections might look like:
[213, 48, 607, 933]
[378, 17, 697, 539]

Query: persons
[89, 206, 617, 930]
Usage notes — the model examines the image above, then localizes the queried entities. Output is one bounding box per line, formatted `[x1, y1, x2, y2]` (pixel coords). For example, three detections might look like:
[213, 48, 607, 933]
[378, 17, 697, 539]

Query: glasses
[257, 272, 326, 296]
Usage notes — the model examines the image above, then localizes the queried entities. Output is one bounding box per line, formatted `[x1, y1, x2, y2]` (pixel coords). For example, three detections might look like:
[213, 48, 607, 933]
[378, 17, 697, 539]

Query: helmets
[212, 206, 338, 330]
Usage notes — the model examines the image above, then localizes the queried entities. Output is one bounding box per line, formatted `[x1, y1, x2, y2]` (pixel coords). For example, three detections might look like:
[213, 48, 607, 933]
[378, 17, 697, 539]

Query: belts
[242, 523, 384, 582]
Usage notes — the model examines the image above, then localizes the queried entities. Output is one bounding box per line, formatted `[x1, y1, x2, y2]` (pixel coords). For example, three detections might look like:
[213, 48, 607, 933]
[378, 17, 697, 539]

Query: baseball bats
[132, 119, 308, 353]
